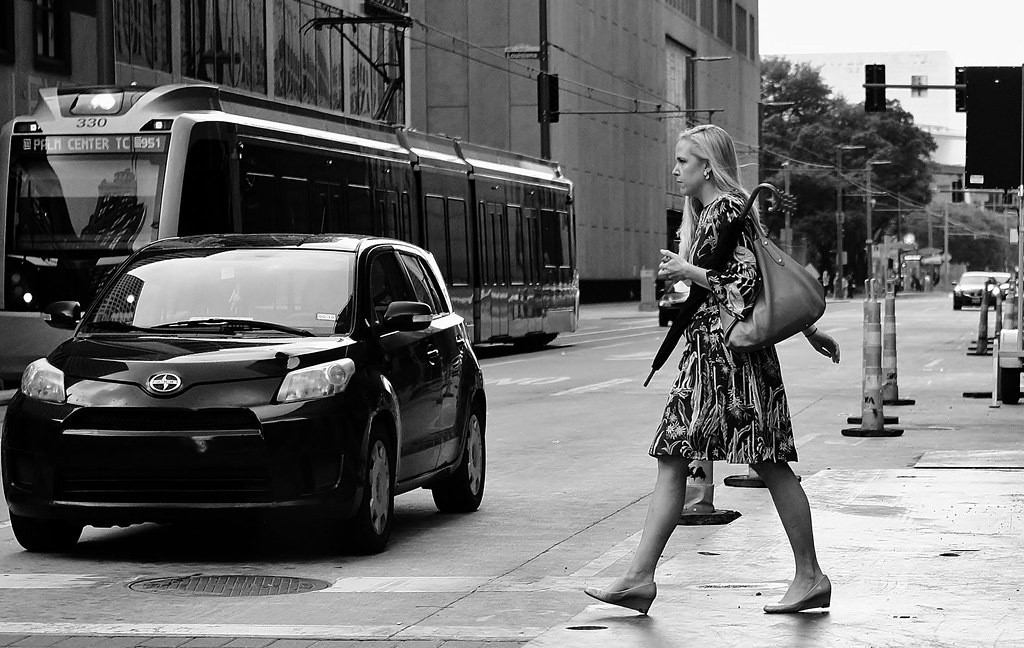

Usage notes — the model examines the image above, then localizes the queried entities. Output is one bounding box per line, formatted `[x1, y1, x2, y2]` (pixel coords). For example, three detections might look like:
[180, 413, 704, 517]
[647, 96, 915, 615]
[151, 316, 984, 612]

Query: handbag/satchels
[712, 192, 826, 354]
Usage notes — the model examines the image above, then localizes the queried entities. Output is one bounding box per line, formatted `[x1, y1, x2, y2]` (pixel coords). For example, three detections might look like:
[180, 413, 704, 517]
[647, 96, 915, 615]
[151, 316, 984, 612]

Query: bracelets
[805, 328, 818, 337]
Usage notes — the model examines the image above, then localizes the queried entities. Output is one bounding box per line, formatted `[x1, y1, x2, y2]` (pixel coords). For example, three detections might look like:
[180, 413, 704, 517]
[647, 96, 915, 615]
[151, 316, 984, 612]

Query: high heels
[584, 582, 657, 614]
[763, 574, 832, 614]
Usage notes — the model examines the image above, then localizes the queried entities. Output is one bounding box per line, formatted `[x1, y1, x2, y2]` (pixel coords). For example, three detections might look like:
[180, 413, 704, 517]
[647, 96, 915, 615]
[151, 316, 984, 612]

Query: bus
[0, 14, 582, 376]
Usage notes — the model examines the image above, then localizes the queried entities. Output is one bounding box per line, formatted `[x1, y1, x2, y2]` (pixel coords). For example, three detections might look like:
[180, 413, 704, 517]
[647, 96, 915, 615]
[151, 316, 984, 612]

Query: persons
[584, 124, 841, 616]
[826, 271, 856, 298]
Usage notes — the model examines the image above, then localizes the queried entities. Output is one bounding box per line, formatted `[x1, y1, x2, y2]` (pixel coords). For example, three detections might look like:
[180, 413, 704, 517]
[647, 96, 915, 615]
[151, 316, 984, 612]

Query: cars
[989, 271, 1011, 302]
[951, 270, 1002, 310]
[1, 228, 489, 558]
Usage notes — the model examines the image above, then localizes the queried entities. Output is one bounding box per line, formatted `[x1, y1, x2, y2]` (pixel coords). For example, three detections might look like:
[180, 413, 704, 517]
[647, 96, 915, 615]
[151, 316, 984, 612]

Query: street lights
[755, 100, 795, 225]
[682, 54, 733, 127]
[864, 160, 893, 294]
[834, 143, 867, 299]
